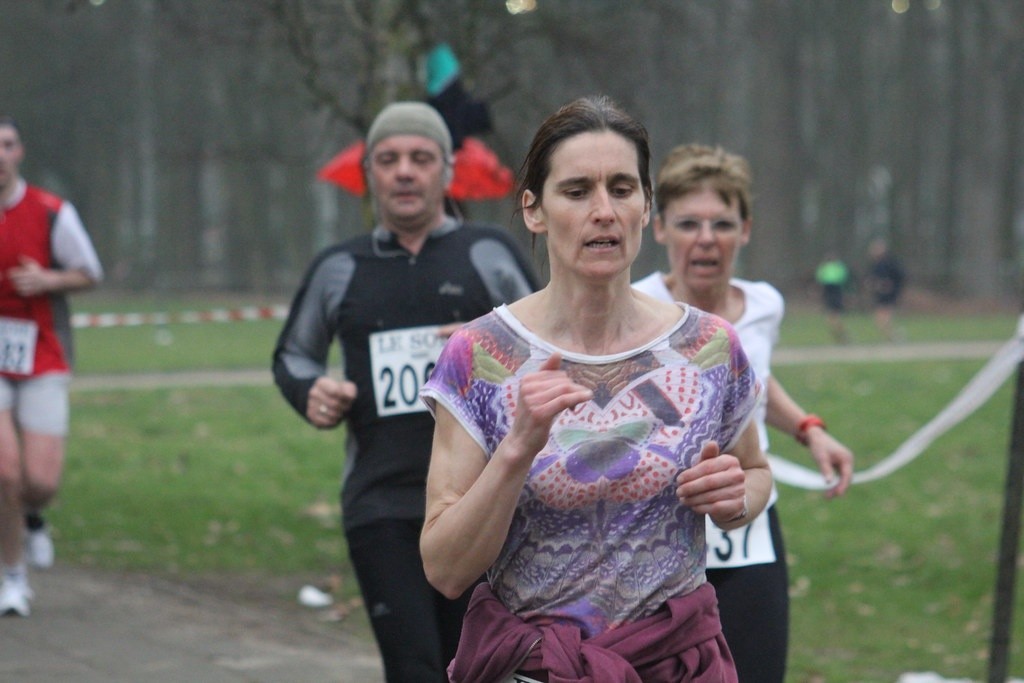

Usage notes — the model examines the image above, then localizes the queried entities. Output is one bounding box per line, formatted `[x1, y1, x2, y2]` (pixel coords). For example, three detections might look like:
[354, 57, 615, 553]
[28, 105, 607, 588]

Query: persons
[867, 239, 904, 338]
[815, 245, 854, 345]
[630, 145, 854, 683]
[417, 94, 774, 683]
[0, 114, 105, 617]
[270, 101, 541, 683]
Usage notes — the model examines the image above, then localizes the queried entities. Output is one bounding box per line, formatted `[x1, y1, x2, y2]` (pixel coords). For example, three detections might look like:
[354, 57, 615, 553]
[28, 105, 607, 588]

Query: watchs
[729, 495, 751, 522]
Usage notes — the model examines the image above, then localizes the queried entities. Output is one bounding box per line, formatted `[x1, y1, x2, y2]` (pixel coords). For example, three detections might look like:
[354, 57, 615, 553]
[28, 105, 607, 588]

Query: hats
[366, 100, 452, 154]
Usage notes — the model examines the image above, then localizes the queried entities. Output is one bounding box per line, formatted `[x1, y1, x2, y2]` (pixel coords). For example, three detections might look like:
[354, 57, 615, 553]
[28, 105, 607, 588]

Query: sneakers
[22, 526, 54, 568]
[0, 566, 31, 617]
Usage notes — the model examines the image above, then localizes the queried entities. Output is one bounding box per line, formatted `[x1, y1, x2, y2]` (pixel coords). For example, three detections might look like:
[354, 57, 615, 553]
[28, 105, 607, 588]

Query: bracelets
[795, 413, 826, 445]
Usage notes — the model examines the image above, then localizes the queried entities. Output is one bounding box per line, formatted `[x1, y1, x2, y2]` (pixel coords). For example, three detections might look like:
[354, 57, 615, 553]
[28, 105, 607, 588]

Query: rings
[319, 405, 326, 414]
